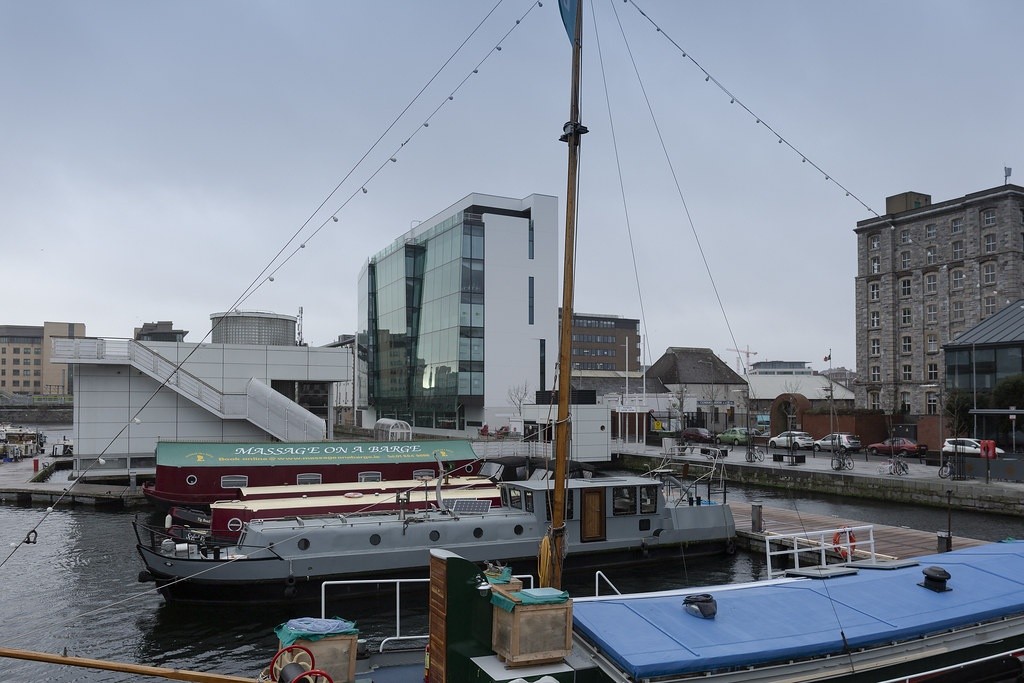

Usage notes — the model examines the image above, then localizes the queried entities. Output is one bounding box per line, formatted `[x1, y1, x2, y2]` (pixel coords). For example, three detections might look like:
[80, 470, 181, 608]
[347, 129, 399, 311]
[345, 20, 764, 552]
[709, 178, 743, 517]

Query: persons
[20, 450, 24, 462]
[481, 424, 488, 437]
[497, 426, 510, 439]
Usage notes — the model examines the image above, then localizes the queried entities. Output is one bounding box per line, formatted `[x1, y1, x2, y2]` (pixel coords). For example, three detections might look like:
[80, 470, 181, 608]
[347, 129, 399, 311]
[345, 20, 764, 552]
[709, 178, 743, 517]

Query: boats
[137, 438, 624, 544]
[130, 445, 740, 612]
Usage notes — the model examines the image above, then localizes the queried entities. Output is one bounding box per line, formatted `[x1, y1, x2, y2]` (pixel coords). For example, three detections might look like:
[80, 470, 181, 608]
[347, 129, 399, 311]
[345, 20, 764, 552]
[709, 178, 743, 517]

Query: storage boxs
[277, 627, 358, 683]
[491, 593, 573, 667]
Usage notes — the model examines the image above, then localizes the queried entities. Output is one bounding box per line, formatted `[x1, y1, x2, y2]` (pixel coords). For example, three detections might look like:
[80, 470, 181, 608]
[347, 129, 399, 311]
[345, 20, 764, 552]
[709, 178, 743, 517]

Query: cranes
[725, 344, 759, 374]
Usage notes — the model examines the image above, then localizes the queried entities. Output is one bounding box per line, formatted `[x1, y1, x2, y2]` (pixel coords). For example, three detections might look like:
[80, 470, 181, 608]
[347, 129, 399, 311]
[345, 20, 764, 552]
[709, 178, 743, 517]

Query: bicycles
[830, 451, 855, 471]
[875, 453, 910, 476]
[744, 445, 765, 462]
[938, 455, 956, 478]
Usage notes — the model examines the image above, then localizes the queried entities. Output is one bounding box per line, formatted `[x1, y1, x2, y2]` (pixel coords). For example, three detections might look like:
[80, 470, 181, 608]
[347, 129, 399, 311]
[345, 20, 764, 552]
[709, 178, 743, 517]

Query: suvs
[811, 431, 862, 455]
[942, 436, 1005, 458]
[768, 431, 815, 451]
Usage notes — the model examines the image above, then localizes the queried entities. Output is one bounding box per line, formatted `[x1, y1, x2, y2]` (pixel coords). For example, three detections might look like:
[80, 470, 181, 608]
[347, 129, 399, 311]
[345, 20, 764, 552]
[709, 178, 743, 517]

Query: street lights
[673, 389, 686, 431]
[934, 385, 944, 475]
[698, 359, 715, 436]
[736, 389, 752, 458]
[822, 383, 834, 467]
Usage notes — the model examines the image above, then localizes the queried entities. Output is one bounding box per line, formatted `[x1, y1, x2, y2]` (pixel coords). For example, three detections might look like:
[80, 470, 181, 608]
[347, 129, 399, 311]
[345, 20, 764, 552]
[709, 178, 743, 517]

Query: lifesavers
[832, 526, 856, 557]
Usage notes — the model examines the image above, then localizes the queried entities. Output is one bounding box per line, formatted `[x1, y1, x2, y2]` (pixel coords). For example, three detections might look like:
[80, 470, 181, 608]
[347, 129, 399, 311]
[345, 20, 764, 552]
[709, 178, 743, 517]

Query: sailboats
[0, 0, 1024, 683]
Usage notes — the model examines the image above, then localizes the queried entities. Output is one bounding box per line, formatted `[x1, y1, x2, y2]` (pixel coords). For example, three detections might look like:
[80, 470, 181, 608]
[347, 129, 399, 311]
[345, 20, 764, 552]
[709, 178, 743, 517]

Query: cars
[867, 437, 928, 458]
[680, 427, 715, 443]
[715, 427, 770, 446]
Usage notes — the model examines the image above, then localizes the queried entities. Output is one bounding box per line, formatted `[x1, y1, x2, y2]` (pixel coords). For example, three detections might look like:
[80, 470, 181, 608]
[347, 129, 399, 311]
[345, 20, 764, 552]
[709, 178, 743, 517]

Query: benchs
[773, 454, 806, 463]
[700, 448, 728, 456]
